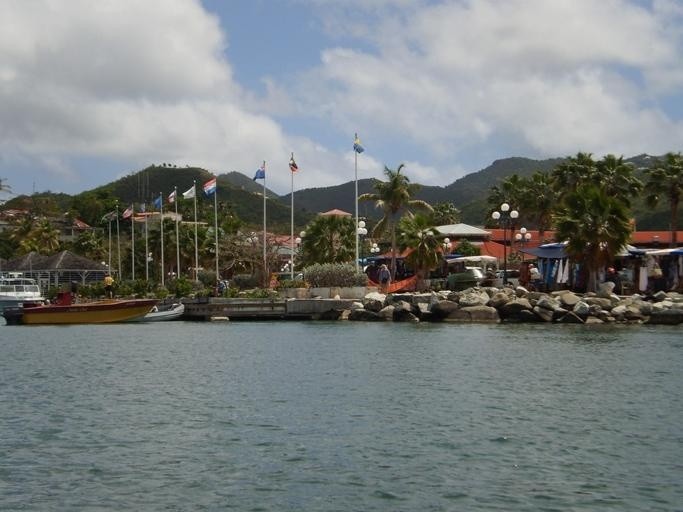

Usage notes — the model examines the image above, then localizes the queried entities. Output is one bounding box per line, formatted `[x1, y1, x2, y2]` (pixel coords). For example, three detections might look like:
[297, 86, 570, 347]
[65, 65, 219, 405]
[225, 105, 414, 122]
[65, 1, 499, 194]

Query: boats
[120, 303, 185, 323]
[0, 276, 42, 312]
[0, 298, 162, 325]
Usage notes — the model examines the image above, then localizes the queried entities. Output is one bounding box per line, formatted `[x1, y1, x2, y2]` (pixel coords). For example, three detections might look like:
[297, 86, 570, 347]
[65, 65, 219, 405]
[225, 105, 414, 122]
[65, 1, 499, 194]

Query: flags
[352, 134, 364, 153]
[122, 204, 133, 219]
[101, 209, 118, 222]
[153, 197, 160, 209]
[252, 162, 264, 180]
[288, 156, 297, 171]
[181, 185, 195, 200]
[167, 190, 175, 203]
[138, 203, 145, 213]
[202, 178, 216, 196]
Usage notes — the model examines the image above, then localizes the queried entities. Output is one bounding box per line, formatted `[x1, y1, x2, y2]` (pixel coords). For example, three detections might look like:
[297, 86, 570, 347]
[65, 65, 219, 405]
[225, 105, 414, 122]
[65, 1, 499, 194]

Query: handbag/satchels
[382, 277, 387, 283]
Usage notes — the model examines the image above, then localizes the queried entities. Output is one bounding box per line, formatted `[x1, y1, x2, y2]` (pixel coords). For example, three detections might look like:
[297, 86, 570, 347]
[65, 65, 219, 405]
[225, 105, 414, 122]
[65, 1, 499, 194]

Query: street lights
[296, 230, 306, 268]
[492, 203, 519, 284]
[370, 243, 381, 266]
[443, 237, 452, 258]
[515, 227, 532, 261]
[358, 220, 368, 274]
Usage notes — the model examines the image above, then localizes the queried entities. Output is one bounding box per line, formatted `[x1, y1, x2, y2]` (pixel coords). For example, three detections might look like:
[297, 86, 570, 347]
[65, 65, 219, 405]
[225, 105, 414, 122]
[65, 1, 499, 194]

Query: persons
[527, 263, 543, 292]
[216, 278, 224, 294]
[376, 263, 390, 294]
[102, 272, 114, 298]
[599, 267, 623, 296]
